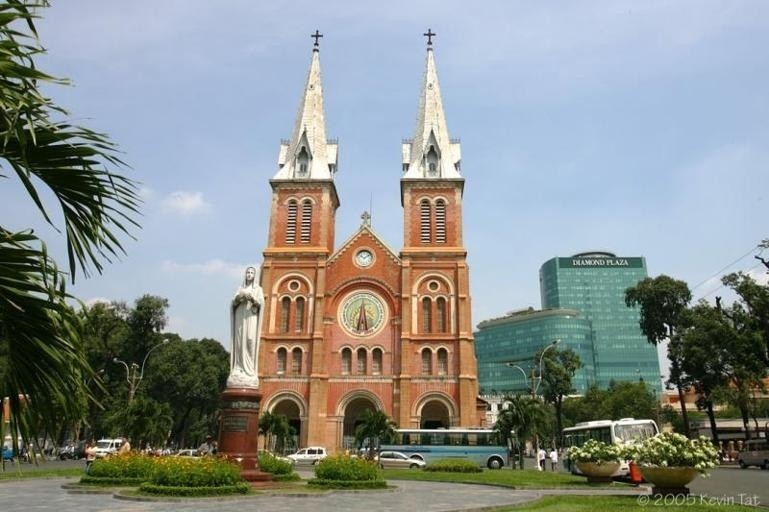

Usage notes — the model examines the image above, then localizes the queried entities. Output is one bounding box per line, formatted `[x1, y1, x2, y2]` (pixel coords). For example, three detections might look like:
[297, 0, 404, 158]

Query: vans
[96, 439, 123, 459]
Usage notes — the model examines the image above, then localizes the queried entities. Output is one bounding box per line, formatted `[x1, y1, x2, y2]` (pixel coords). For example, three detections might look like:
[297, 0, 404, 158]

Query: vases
[637, 463, 699, 497]
[576, 461, 619, 484]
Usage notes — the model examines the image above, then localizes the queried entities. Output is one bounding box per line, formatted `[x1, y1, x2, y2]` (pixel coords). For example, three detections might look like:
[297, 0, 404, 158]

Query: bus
[561, 417, 668, 483]
[377, 429, 520, 470]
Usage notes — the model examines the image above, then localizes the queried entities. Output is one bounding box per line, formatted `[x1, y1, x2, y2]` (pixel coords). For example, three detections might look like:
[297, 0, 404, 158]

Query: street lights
[75, 369, 105, 445]
[505, 339, 561, 448]
[112, 339, 169, 407]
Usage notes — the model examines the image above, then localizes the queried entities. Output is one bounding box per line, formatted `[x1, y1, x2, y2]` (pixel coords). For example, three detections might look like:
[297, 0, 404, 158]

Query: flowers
[566, 432, 622, 464]
[619, 429, 721, 479]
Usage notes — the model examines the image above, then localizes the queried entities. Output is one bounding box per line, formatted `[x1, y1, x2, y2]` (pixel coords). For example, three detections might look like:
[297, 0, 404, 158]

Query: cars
[374, 451, 426, 469]
[287, 447, 327, 466]
[1, 445, 17, 462]
[170, 449, 204, 461]
[256, 450, 293, 469]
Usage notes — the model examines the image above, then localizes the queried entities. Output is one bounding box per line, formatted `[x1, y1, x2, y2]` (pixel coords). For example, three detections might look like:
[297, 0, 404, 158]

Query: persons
[550, 449, 558, 472]
[69, 434, 217, 474]
[538, 446, 546, 471]
[226, 267, 265, 389]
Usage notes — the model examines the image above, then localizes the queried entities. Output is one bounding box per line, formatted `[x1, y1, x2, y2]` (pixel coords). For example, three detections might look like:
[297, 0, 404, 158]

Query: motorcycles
[59, 450, 79, 460]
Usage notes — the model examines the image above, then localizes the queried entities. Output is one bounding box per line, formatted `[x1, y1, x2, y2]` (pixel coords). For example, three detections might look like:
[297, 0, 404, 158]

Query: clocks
[352, 246, 377, 270]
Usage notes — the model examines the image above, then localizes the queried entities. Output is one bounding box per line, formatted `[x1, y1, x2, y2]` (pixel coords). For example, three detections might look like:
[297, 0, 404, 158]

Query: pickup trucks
[735, 439, 769, 470]
